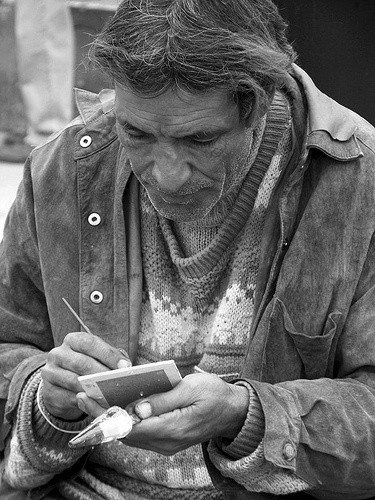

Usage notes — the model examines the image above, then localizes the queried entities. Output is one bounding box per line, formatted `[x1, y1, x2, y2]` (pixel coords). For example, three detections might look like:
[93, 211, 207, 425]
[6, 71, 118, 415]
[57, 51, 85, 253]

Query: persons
[0, 0, 375, 500]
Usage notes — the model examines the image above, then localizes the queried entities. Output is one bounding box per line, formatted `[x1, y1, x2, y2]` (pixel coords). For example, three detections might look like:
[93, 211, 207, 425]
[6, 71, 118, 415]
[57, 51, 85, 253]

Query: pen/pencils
[62, 297, 91, 334]
